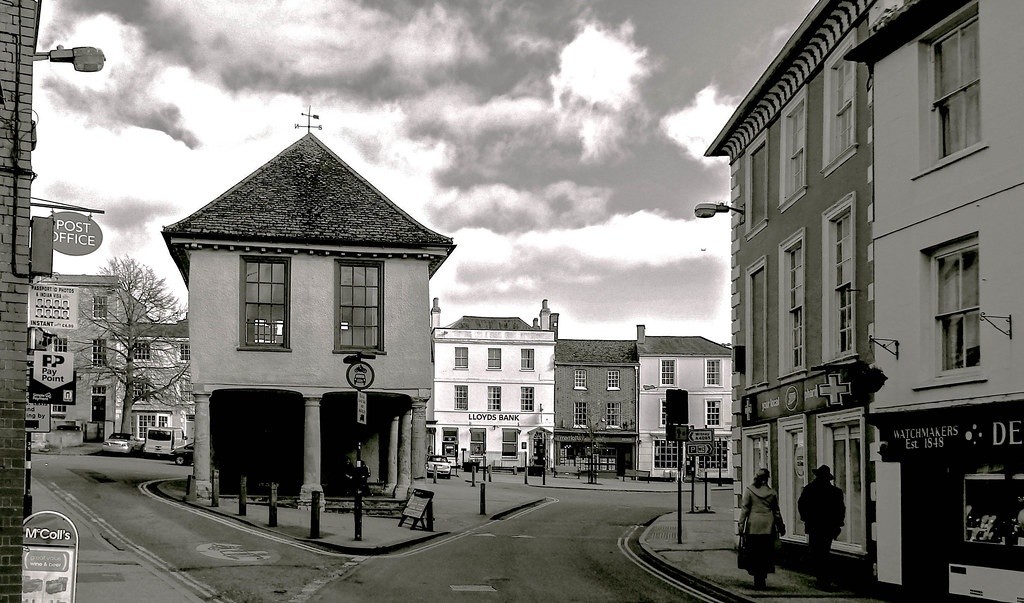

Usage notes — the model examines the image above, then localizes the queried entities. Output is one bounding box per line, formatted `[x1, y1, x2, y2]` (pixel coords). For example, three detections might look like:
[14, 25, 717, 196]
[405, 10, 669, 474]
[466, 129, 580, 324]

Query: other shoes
[808, 580, 829, 591]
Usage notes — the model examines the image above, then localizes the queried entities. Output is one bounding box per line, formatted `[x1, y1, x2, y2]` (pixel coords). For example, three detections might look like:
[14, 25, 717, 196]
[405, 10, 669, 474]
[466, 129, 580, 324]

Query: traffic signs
[688, 429, 714, 442]
[686, 442, 713, 455]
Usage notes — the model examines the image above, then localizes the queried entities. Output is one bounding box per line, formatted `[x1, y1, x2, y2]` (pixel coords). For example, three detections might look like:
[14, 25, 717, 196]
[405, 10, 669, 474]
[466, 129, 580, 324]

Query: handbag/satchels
[737, 534, 747, 569]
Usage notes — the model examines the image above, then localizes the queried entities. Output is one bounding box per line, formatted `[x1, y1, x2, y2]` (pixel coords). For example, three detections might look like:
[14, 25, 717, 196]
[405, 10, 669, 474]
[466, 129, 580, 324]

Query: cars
[168, 442, 194, 465]
[103, 433, 140, 454]
[427, 455, 452, 479]
[142, 427, 188, 457]
[56, 425, 86, 442]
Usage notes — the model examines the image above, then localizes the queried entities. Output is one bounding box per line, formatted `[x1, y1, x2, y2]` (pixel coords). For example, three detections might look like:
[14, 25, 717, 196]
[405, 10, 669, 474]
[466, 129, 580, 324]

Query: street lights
[343, 350, 376, 541]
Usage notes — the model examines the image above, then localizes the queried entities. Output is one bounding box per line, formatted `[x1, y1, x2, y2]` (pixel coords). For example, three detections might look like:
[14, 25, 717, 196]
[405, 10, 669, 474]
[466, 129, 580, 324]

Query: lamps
[342, 351, 376, 365]
[694, 201, 745, 225]
[601, 418, 605, 422]
[33, 44, 106, 73]
[493, 425, 496, 430]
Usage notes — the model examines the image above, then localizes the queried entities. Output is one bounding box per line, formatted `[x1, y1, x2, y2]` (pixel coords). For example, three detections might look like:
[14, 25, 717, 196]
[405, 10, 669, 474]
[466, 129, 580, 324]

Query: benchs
[623, 469, 650, 484]
[553, 466, 581, 479]
[487, 466, 517, 475]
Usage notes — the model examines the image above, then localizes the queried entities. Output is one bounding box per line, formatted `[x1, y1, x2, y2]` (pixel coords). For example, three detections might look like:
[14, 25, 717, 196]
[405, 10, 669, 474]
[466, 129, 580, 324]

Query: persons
[798, 465, 846, 587]
[344, 456, 374, 497]
[737, 468, 786, 589]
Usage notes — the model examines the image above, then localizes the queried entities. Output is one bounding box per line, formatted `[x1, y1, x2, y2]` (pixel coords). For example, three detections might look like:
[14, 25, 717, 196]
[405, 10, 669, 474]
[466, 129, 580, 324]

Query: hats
[812, 465, 833, 480]
